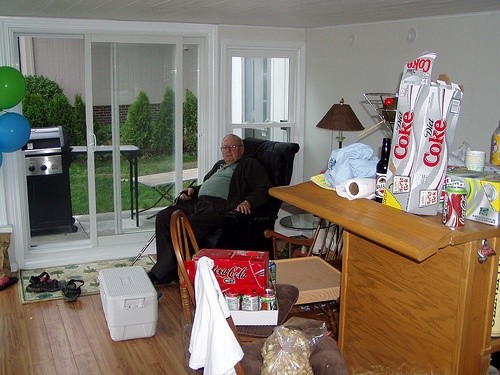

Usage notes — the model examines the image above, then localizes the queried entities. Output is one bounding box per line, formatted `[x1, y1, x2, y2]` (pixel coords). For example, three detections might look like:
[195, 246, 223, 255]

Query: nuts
[259, 326, 314, 375]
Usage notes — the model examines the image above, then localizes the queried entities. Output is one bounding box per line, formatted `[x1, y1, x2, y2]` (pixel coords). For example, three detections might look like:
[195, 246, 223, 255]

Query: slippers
[0, 277, 18, 291]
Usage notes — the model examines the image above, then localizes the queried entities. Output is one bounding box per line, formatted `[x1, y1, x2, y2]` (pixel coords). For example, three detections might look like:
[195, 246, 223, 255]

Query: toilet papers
[335, 177, 376, 201]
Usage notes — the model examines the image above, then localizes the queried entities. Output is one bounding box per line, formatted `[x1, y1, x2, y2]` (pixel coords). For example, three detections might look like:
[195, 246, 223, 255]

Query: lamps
[316, 97, 365, 271]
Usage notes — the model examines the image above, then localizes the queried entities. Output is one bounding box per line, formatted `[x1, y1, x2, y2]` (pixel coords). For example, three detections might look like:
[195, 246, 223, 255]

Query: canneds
[223, 260, 277, 311]
[442, 186, 467, 228]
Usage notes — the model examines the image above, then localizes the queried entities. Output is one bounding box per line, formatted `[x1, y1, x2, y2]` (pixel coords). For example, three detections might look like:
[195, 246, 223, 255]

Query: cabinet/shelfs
[268, 181, 500, 375]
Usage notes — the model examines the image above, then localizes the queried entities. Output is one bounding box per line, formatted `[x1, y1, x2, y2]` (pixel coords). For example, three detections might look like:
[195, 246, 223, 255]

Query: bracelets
[244, 199, 251, 208]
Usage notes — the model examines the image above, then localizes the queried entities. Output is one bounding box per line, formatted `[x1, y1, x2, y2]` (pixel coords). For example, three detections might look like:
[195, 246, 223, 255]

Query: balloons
[0, 66, 31, 167]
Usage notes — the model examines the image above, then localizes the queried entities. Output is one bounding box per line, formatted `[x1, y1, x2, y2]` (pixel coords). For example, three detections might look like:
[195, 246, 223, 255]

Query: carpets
[18, 254, 175, 303]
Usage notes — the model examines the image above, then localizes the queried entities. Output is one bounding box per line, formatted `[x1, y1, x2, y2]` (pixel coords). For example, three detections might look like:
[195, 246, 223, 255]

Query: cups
[464, 151, 486, 172]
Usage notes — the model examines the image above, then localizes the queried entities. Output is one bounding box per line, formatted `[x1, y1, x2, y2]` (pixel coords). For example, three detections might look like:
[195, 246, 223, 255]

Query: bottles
[490, 122, 500, 170]
[375, 137, 391, 203]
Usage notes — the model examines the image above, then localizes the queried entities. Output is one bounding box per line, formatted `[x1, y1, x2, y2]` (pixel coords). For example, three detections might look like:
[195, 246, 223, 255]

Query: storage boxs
[182, 248, 269, 298]
[231, 297, 279, 326]
[98, 266, 158, 342]
[382, 52, 500, 228]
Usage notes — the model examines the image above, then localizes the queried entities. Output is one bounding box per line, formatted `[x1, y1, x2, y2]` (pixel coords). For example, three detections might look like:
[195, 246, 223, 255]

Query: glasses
[221, 144, 241, 150]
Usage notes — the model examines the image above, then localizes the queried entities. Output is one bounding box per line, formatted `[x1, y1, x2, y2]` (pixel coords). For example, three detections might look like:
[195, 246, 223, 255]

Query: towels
[188, 255, 245, 375]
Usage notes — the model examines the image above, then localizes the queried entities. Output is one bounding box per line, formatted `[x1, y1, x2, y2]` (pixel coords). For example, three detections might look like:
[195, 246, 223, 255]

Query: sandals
[61, 279, 84, 302]
[26, 272, 66, 292]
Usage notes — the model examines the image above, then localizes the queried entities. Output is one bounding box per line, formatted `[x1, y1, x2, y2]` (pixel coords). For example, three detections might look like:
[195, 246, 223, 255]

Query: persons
[147, 134, 273, 285]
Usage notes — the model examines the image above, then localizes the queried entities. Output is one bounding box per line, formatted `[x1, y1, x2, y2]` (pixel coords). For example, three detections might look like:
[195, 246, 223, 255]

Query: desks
[280, 215, 337, 260]
[69, 144, 140, 227]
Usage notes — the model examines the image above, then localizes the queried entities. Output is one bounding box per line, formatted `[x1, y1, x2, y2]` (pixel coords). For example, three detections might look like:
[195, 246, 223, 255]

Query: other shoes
[159, 279, 173, 284]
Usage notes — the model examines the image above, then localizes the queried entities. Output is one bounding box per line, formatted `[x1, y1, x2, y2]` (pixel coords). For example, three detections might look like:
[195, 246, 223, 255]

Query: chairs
[183, 138, 300, 259]
[134, 168, 199, 219]
[169, 209, 349, 375]
